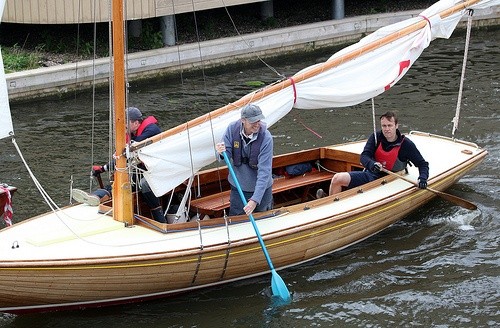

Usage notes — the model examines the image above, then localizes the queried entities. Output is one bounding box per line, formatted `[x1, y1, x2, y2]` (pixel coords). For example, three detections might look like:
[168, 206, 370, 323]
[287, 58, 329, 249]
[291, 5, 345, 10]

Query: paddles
[220, 147, 290, 299]
[381, 166, 477, 210]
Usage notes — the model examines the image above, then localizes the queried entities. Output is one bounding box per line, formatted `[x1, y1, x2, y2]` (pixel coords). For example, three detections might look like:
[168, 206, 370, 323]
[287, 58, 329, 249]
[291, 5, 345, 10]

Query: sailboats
[1, 0, 489, 314]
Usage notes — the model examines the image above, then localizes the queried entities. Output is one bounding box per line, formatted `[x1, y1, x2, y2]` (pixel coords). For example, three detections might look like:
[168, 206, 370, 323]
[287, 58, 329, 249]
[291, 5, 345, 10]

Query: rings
[247, 209, 250, 213]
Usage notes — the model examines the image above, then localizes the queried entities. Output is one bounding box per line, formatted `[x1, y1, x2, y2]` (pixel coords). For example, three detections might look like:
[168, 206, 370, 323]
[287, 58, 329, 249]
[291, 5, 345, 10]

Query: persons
[71, 106, 166, 223]
[329, 111, 429, 196]
[215, 103, 274, 216]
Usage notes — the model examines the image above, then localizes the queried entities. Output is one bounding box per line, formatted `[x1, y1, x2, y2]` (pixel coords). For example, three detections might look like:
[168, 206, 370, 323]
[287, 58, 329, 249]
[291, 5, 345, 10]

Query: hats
[240, 103, 266, 124]
[125, 107, 144, 120]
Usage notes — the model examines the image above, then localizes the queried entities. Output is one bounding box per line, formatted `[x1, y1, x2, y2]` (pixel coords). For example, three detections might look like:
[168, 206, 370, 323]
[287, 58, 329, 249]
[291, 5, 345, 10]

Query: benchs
[189, 169, 336, 217]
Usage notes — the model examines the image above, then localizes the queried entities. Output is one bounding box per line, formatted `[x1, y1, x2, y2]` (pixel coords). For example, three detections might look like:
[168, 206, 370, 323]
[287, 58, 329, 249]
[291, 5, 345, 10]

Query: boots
[150, 206, 167, 224]
[71, 188, 111, 206]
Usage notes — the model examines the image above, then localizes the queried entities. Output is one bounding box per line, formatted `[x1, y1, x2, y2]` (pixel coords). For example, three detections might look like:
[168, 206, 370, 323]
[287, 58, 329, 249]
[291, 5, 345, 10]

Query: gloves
[417, 178, 428, 189]
[92, 165, 104, 175]
[371, 162, 382, 173]
